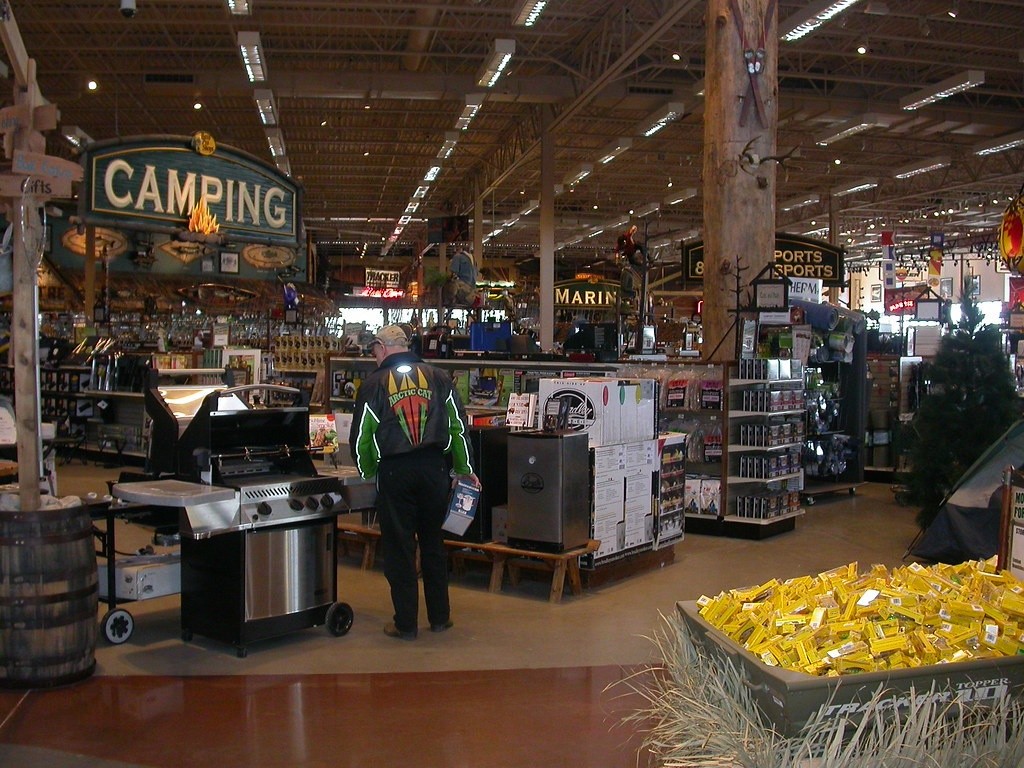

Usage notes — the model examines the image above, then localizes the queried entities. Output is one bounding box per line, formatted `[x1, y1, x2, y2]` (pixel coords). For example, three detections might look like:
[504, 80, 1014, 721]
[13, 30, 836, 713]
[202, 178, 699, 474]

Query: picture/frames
[219, 251, 240, 275]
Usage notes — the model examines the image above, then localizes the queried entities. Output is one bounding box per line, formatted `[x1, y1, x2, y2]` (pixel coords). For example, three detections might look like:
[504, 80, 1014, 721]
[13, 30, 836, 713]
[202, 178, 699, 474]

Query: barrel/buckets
[0, 494, 101, 693]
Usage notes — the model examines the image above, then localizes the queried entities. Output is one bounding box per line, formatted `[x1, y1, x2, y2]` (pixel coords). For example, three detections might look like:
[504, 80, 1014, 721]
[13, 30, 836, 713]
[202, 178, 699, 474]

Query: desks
[337, 524, 601, 604]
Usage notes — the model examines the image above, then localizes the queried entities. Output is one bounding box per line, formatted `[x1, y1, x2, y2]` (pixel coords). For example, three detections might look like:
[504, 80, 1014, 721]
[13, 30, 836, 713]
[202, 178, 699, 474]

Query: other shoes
[431, 620, 453, 633]
[384, 623, 416, 641]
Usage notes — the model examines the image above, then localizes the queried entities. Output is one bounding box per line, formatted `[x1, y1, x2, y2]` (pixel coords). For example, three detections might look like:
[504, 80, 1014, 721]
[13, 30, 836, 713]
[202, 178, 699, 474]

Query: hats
[367, 324, 408, 346]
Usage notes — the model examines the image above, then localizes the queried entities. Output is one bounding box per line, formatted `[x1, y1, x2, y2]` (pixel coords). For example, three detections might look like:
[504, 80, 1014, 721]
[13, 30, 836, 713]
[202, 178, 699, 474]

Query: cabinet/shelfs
[329, 354, 618, 411]
[622, 359, 806, 538]
[805, 430, 870, 503]
[58, 390, 148, 465]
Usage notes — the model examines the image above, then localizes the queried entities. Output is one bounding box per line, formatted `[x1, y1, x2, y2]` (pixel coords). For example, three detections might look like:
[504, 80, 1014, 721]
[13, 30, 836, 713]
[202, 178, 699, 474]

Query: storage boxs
[271, 335, 339, 369]
[0, 369, 94, 418]
[685, 473, 721, 517]
[675, 598, 1024, 753]
[446, 367, 685, 566]
[736, 359, 804, 518]
[866, 360, 892, 466]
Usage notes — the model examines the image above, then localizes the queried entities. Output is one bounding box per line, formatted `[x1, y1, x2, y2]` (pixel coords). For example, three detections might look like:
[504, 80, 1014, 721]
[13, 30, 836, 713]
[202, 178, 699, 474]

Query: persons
[491, 289, 518, 308]
[347, 323, 483, 641]
[615, 225, 654, 271]
[450, 250, 479, 289]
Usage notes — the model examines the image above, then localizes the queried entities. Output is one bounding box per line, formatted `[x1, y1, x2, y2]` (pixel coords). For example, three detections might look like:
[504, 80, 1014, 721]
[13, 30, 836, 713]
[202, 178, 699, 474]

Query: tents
[899, 417, 1024, 566]
